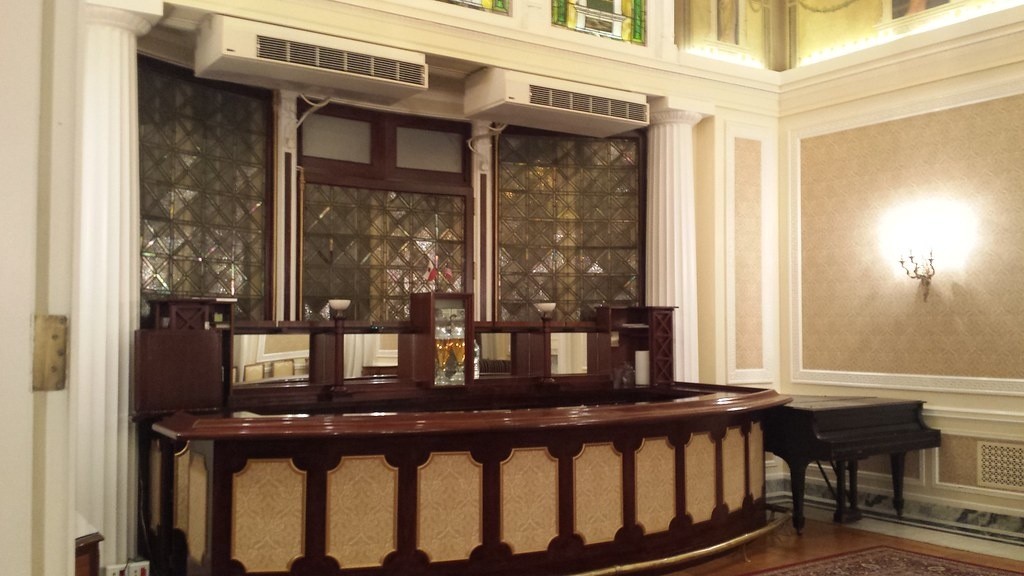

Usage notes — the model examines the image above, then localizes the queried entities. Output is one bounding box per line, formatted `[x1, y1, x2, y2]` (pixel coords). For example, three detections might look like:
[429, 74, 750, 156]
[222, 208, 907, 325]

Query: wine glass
[450, 327, 465, 382]
[435, 328, 452, 385]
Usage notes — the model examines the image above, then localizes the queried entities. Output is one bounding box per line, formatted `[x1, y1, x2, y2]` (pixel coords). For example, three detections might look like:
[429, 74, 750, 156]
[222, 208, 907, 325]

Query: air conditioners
[193, 11, 428, 105]
[464, 67, 649, 137]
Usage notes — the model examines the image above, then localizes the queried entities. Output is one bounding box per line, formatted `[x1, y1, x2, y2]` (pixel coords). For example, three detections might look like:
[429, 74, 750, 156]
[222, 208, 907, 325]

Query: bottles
[474, 340, 480, 376]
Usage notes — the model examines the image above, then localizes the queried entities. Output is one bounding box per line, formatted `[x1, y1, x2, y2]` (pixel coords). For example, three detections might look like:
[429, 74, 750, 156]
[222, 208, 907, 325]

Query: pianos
[762, 393, 941, 536]
